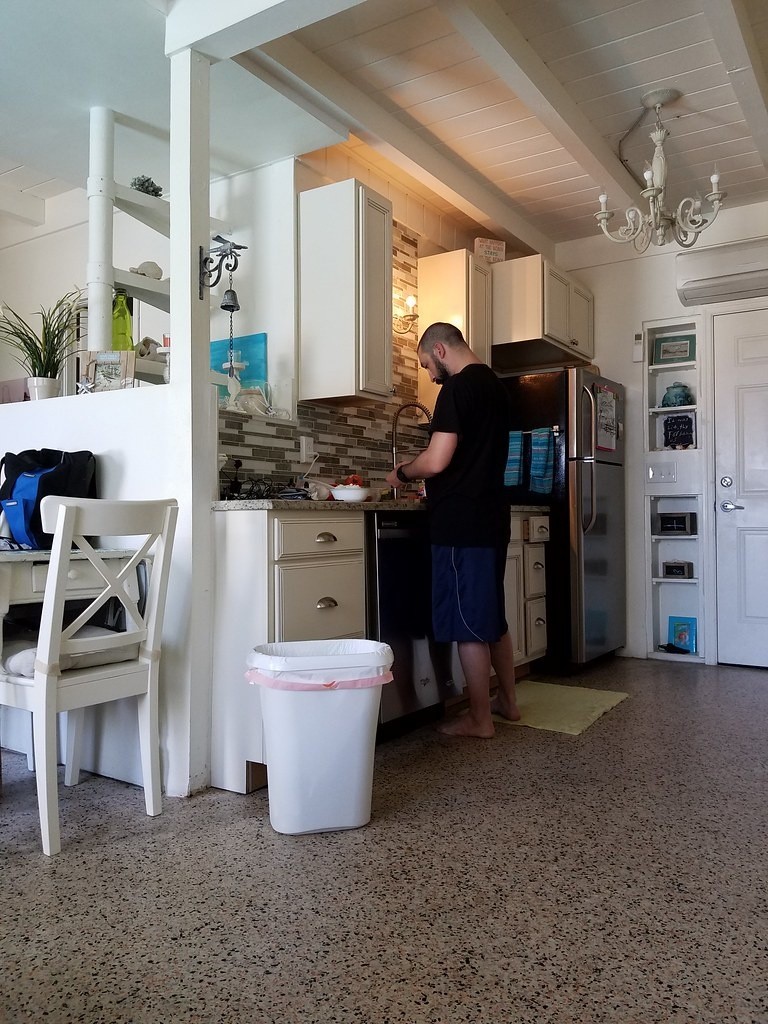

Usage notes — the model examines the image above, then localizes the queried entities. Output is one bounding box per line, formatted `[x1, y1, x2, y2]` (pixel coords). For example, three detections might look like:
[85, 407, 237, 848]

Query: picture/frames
[660, 411, 697, 449]
[654, 334, 696, 365]
[668, 615, 697, 654]
[84, 350, 135, 393]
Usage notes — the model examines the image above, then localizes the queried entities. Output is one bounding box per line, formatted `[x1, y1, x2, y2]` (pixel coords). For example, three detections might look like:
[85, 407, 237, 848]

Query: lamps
[594, 87, 729, 255]
[391, 295, 420, 335]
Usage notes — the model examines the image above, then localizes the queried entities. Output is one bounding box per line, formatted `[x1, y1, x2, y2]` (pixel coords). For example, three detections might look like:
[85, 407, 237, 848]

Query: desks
[0, 550, 141, 775]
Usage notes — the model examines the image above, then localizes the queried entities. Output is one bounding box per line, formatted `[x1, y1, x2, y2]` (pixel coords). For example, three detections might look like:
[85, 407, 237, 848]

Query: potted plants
[0, 284, 88, 401]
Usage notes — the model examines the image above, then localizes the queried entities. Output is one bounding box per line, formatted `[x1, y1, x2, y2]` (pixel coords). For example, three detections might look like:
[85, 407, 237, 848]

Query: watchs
[397, 466, 412, 484]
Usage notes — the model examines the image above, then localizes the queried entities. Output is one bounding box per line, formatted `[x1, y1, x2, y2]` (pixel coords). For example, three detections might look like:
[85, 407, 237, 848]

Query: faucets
[390, 401, 433, 500]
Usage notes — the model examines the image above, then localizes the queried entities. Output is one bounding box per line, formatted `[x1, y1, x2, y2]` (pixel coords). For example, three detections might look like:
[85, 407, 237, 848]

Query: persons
[385, 322, 521, 738]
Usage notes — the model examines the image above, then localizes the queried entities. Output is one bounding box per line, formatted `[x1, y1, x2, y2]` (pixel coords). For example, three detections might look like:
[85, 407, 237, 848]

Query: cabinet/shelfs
[417, 248, 493, 426]
[84, 107, 234, 388]
[641, 314, 705, 457]
[212, 511, 371, 795]
[489, 252, 595, 375]
[645, 492, 706, 665]
[452, 513, 550, 707]
[297, 176, 394, 410]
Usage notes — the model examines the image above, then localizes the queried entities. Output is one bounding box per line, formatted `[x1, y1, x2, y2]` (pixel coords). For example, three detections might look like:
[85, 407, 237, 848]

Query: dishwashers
[365, 511, 470, 736]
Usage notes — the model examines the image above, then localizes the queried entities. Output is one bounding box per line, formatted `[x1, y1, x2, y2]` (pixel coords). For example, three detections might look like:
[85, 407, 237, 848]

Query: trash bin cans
[246, 639, 395, 834]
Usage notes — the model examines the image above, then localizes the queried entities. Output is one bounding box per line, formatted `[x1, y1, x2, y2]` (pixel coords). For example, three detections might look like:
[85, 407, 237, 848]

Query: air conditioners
[675, 237, 768, 307]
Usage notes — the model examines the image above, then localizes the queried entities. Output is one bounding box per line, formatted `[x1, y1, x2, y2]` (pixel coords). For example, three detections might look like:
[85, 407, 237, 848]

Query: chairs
[0, 494, 179, 855]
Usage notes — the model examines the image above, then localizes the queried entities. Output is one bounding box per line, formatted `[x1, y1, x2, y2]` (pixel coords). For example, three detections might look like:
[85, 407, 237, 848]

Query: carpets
[459, 679, 629, 736]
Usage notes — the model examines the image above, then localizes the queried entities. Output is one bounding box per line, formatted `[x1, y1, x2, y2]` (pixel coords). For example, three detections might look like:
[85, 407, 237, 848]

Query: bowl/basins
[330, 488, 369, 502]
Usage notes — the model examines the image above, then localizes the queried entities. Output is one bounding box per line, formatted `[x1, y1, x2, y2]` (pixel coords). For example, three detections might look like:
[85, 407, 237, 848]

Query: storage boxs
[655, 513, 697, 535]
[474, 237, 506, 264]
[663, 562, 694, 579]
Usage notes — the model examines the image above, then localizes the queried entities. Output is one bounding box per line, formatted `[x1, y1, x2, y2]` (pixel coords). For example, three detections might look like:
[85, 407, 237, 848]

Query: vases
[662, 385, 693, 408]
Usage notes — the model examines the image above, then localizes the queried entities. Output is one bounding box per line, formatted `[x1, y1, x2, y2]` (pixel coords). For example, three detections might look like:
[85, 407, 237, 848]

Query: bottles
[113, 290, 132, 351]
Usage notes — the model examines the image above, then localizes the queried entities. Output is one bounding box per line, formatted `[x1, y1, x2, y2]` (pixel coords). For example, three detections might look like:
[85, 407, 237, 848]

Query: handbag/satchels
[0, 449, 96, 550]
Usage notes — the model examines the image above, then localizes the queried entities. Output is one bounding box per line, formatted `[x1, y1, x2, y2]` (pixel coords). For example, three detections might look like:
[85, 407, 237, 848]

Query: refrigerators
[502, 368, 626, 675]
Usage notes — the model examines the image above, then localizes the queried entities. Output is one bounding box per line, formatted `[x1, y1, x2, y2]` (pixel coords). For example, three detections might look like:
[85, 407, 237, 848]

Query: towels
[529, 427, 555, 494]
[504, 430, 524, 486]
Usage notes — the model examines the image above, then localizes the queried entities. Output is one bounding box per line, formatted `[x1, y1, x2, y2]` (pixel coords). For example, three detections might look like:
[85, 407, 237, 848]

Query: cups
[163, 333, 170, 347]
